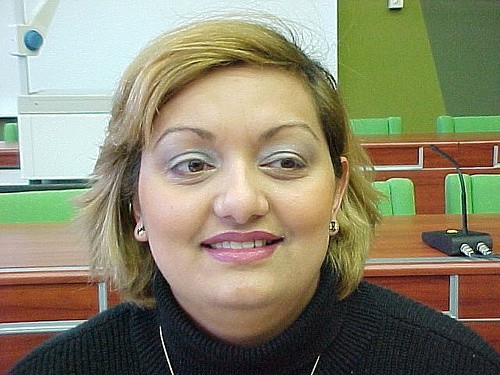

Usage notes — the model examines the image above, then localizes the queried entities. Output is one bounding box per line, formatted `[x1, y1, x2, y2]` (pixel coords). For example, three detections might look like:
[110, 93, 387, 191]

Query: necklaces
[157, 324, 323, 375]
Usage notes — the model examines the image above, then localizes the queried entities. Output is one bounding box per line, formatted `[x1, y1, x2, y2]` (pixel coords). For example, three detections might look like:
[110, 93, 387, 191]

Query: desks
[0, 214, 500, 375]
[351, 132, 500, 216]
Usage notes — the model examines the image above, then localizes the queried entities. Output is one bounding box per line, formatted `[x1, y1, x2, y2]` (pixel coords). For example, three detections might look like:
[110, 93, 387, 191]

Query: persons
[1, 5, 500, 375]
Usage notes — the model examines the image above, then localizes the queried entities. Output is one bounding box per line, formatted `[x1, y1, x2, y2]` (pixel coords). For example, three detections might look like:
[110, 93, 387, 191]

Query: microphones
[421, 144, 494, 257]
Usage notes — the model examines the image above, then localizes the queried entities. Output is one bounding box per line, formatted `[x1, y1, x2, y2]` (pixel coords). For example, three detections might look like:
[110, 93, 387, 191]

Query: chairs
[347, 114, 500, 216]
